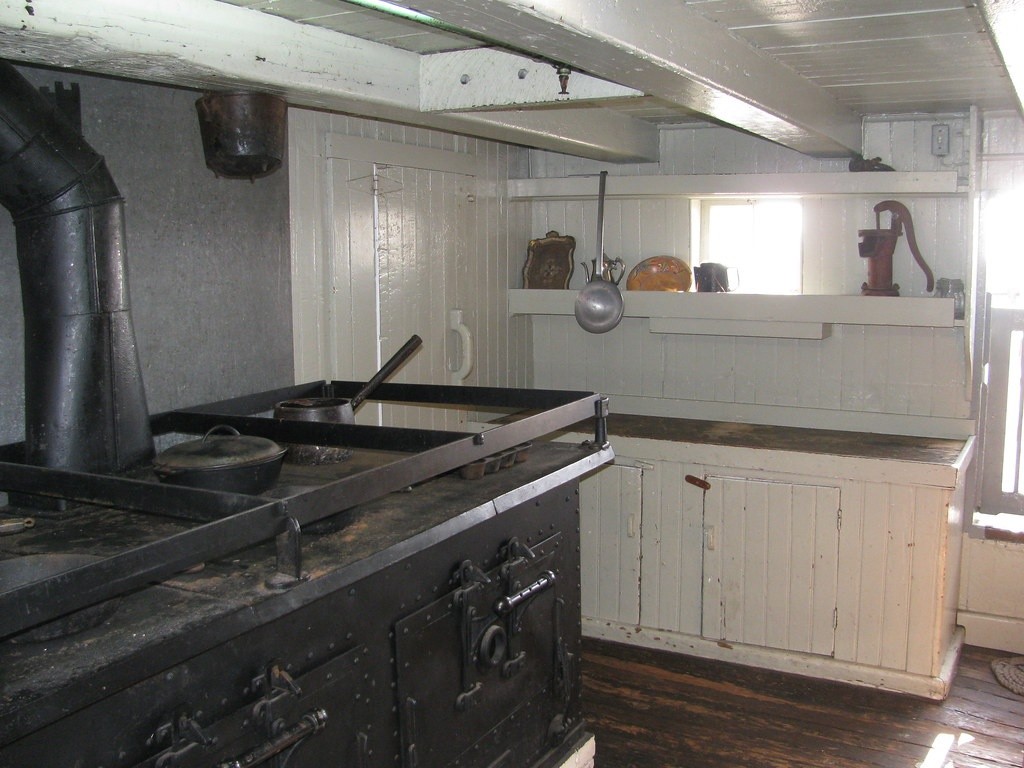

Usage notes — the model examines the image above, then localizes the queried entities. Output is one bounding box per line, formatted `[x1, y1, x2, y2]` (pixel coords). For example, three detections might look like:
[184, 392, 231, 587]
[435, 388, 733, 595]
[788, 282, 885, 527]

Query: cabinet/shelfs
[475, 407, 976, 702]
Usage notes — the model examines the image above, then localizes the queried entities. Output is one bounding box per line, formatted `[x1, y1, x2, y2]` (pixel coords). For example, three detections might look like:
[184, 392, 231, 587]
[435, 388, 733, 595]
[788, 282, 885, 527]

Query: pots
[151, 425, 290, 494]
[273, 334, 423, 424]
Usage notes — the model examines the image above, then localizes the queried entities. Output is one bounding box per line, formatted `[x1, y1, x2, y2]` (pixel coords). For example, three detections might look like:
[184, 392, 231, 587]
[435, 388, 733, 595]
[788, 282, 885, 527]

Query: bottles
[933, 278, 965, 319]
